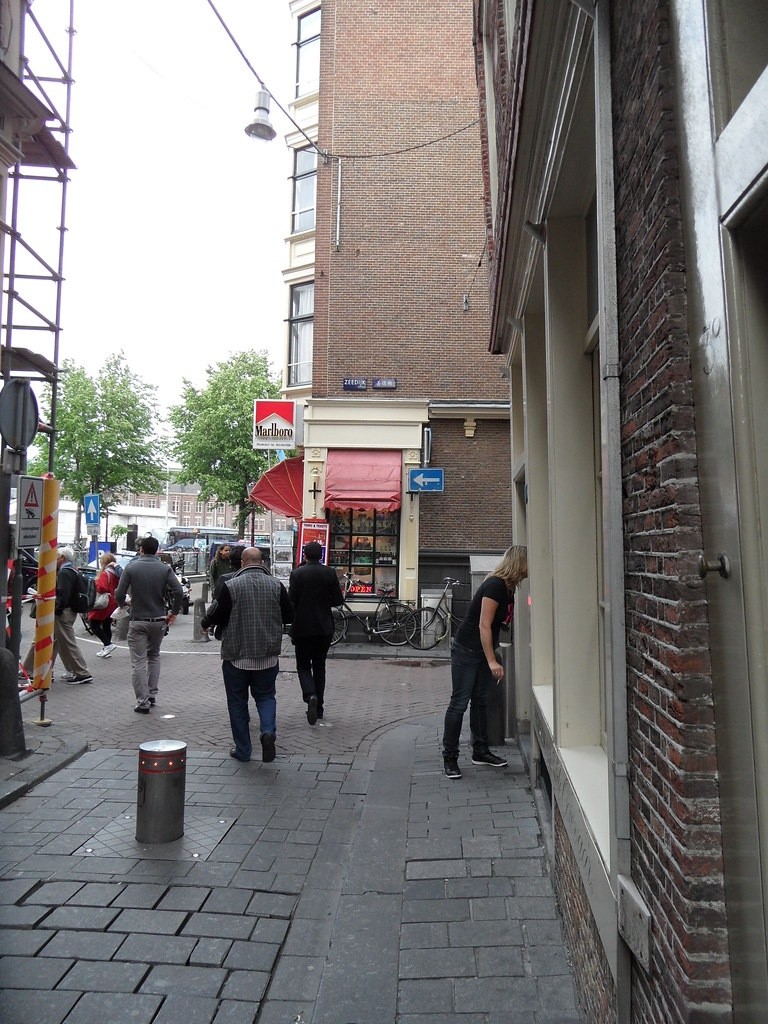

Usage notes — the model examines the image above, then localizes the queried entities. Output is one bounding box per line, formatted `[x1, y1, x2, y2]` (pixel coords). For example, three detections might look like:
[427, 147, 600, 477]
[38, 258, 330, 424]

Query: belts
[129, 618, 165, 622]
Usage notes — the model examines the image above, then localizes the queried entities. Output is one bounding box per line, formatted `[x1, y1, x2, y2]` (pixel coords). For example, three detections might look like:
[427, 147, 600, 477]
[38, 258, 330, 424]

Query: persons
[442, 545, 528, 779]
[201, 548, 290, 763]
[289, 543, 344, 725]
[115, 537, 183, 714]
[18, 547, 92, 685]
[91, 553, 125, 659]
[201, 545, 236, 642]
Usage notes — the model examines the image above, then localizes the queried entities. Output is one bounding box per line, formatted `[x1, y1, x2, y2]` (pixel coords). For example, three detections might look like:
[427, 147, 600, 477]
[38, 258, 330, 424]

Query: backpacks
[62, 565, 96, 613]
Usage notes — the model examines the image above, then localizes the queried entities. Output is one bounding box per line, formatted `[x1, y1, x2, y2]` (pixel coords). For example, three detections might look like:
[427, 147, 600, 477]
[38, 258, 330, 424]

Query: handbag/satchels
[92, 592, 111, 609]
[110, 606, 129, 619]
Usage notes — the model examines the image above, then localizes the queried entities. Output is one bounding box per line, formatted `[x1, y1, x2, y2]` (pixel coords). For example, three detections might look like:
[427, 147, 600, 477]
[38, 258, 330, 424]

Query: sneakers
[444, 759, 462, 778]
[472, 750, 508, 766]
[67, 674, 93, 684]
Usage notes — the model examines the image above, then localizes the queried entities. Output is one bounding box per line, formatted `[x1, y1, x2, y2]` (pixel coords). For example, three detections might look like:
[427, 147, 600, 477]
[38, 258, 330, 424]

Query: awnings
[249, 449, 402, 519]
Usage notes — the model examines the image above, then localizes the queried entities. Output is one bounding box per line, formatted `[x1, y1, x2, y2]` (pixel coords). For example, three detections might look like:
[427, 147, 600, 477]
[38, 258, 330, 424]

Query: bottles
[334, 551, 346, 564]
[379, 552, 392, 564]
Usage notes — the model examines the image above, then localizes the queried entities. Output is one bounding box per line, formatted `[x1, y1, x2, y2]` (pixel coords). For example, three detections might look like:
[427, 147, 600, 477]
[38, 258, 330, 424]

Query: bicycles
[404, 577, 465, 650]
[331, 572, 419, 647]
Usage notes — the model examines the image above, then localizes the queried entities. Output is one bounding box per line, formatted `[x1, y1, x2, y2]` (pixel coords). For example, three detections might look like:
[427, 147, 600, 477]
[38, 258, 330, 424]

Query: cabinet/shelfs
[326, 507, 400, 598]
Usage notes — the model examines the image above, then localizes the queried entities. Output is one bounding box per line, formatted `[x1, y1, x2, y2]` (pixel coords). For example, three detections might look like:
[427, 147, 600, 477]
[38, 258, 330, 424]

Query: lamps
[245, 85, 277, 143]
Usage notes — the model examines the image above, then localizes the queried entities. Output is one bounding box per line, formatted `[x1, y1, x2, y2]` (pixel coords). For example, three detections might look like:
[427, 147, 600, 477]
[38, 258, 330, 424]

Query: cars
[88, 526, 274, 570]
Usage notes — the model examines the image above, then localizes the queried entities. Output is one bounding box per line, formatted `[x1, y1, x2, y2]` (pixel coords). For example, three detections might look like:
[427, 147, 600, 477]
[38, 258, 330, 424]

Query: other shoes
[230, 748, 250, 762]
[134, 705, 149, 714]
[305, 711, 324, 719]
[307, 695, 318, 725]
[96, 648, 112, 658]
[18, 669, 32, 679]
[101, 644, 117, 657]
[261, 733, 276, 762]
[138, 697, 156, 703]
